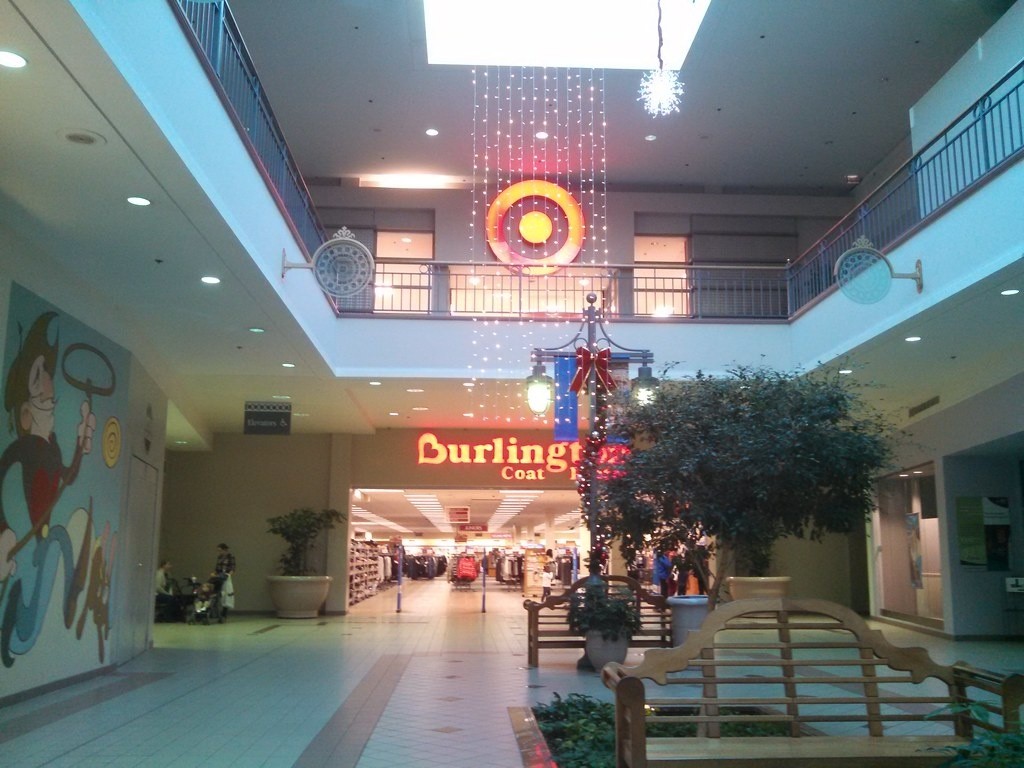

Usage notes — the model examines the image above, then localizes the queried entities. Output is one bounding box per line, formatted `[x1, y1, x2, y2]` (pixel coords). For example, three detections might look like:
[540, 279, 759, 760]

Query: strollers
[183, 574, 225, 625]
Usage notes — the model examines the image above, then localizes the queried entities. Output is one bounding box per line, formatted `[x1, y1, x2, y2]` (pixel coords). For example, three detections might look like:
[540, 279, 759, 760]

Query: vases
[668, 595, 709, 671]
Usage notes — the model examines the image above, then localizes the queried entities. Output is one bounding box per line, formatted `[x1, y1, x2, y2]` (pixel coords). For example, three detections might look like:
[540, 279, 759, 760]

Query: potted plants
[567, 574, 645, 673]
[264, 507, 348, 618]
[726, 546, 794, 618]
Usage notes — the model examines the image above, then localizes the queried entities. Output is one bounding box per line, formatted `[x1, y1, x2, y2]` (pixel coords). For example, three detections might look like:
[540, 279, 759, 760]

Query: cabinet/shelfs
[350, 547, 380, 604]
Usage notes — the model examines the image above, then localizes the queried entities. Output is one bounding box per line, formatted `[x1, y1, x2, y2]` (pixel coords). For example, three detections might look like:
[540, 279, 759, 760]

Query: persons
[192, 583, 213, 613]
[156, 559, 175, 623]
[387, 543, 400, 581]
[209, 542, 236, 623]
[650, 545, 707, 612]
[543, 548, 555, 595]
[541, 565, 553, 603]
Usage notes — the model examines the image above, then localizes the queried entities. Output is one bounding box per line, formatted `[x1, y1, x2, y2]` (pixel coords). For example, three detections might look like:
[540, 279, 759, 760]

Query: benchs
[524, 574, 671, 670]
[600, 595, 1024, 768]
